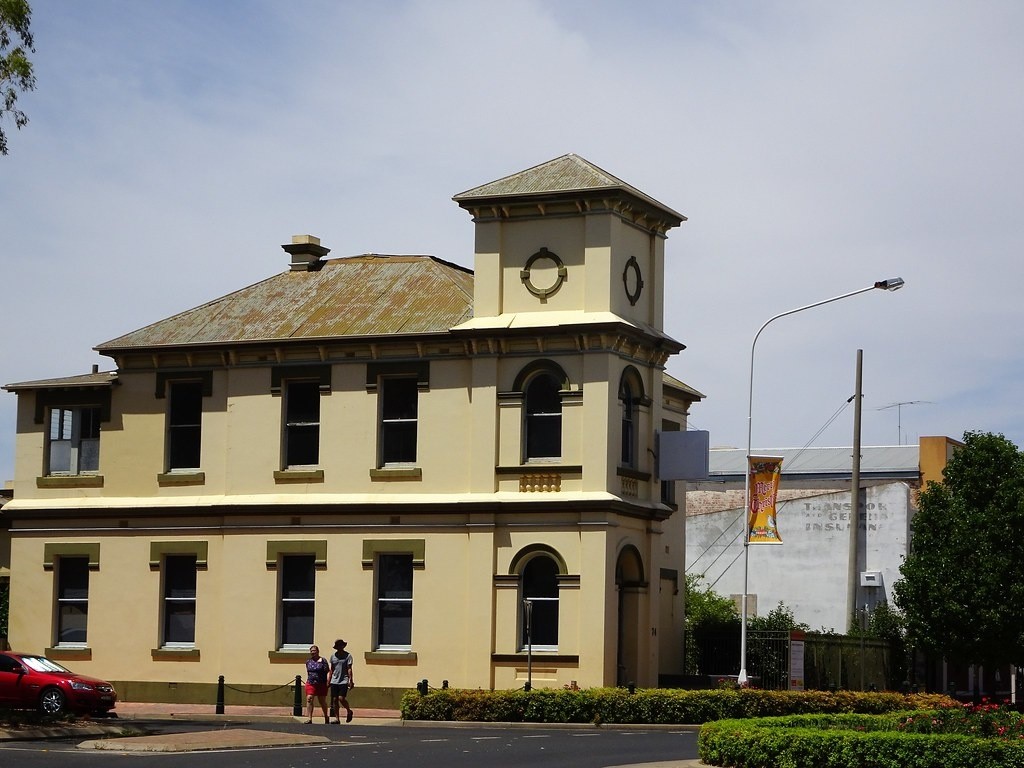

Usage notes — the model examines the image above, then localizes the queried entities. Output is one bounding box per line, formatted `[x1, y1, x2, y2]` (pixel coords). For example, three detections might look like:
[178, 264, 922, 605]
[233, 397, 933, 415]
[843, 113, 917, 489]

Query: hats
[333, 640, 347, 650]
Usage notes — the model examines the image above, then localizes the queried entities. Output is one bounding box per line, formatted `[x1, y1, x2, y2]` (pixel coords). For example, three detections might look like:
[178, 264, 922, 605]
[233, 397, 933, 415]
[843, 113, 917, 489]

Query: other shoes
[304, 717, 329, 724]
[346, 710, 353, 723]
[331, 720, 340, 724]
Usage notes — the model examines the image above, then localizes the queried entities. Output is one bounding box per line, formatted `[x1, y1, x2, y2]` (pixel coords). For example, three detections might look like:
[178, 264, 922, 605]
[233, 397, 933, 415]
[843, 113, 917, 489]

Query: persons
[327, 639, 354, 725]
[305, 645, 328, 726]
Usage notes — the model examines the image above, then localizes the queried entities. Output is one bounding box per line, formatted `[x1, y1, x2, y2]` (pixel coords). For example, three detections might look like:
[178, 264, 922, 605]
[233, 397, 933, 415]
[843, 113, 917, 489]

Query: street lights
[738, 275, 905, 690]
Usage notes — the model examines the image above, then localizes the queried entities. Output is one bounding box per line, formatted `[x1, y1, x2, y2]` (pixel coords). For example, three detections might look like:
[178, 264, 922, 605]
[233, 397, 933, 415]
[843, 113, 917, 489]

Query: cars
[0, 650, 118, 721]
